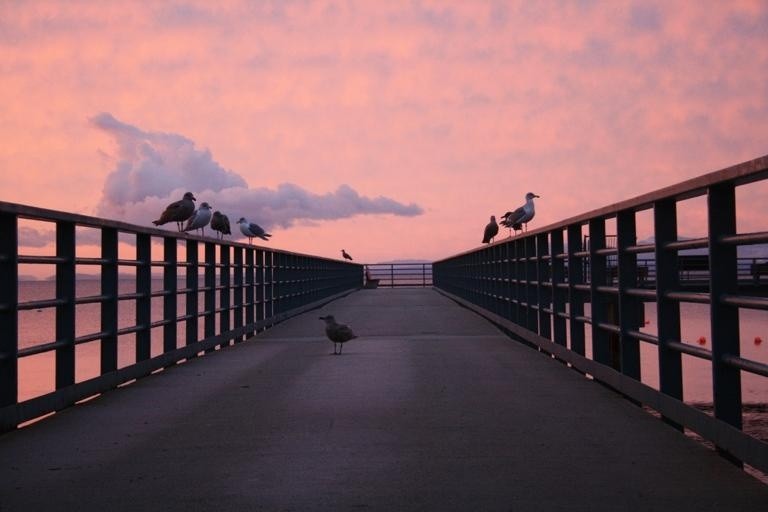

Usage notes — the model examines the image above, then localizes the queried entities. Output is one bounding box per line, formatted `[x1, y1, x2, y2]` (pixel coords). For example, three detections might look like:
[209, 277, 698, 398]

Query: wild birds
[236, 216, 272, 245]
[482, 215, 499, 245]
[340, 249, 353, 261]
[500, 192, 540, 233]
[151, 192, 197, 232]
[318, 314, 359, 355]
[500, 212, 522, 238]
[210, 211, 232, 240]
[183, 202, 213, 237]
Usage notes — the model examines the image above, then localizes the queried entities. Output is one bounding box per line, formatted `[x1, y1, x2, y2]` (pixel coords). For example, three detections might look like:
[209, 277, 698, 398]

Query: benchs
[365, 267, 381, 289]
[606, 265, 649, 282]
